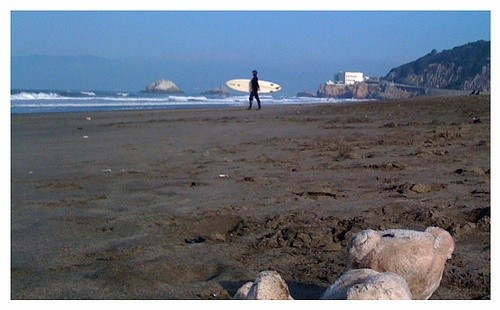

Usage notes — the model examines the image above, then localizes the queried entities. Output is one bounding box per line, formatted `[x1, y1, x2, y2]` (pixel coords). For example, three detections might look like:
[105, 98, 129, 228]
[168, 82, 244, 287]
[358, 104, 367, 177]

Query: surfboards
[225, 79, 282, 94]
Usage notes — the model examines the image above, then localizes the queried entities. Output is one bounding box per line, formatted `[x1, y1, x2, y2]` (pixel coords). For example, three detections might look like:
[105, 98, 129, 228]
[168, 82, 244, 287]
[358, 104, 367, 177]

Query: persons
[246, 70, 262, 110]
